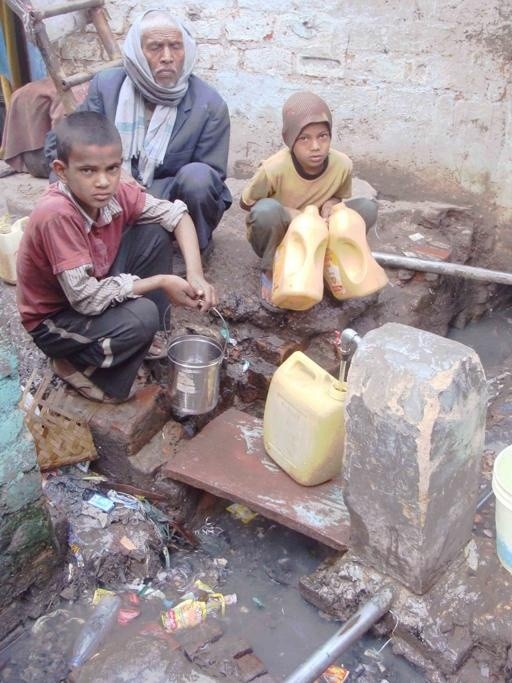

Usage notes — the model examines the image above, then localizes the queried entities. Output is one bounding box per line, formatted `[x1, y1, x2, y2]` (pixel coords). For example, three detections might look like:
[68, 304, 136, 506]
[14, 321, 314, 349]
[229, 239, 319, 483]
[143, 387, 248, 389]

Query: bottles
[159, 593, 238, 634]
[69, 594, 120, 668]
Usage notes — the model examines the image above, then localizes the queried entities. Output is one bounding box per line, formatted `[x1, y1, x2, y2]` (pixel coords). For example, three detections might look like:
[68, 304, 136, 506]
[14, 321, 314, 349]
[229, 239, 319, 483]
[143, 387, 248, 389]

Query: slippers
[46, 357, 137, 404]
[259, 268, 288, 314]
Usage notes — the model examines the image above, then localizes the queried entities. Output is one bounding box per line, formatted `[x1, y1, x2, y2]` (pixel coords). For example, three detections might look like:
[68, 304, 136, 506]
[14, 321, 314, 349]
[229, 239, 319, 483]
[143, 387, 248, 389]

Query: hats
[282, 92, 332, 153]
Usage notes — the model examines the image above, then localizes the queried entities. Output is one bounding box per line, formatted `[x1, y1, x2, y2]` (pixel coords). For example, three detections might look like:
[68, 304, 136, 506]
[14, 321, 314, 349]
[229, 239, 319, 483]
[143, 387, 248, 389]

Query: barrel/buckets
[324, 204, 389, 301]
[262, 351, 349, 486]
[271, 205, 329, 311]
[490, 445, 512, 575]
[0, 216, 31, 284]
[157, 299, 230, 414]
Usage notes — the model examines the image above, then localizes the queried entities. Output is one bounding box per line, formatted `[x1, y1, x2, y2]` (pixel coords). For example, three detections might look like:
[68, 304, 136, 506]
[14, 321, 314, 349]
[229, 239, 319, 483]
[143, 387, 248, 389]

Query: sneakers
[173, 236, 215, 279]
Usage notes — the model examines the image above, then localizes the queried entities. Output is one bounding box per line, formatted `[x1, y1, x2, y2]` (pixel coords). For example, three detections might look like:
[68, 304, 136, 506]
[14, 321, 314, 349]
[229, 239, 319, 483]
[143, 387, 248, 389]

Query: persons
[45, 9, 232, 278]
[239, 91, 379, 313]
[17, 110, 218, 405]
